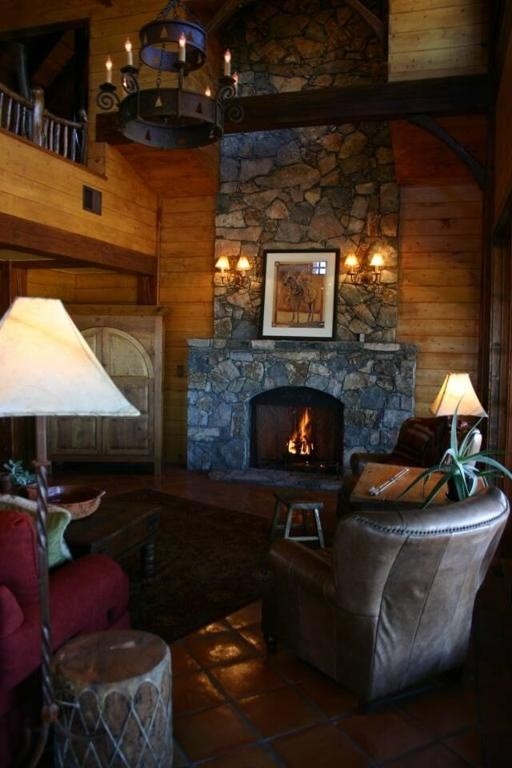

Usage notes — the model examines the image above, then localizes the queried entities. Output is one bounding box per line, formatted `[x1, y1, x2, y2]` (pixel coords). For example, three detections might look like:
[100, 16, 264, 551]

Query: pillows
[0, 493, 72, 571]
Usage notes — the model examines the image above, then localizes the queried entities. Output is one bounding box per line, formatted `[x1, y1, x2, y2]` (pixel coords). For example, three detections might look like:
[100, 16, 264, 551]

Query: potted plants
[395, 396, 512, 510]
[5, 458, 37, 501]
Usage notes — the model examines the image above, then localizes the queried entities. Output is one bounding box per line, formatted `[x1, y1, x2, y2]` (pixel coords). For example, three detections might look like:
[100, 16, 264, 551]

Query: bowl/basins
[45, 484, 107, 521]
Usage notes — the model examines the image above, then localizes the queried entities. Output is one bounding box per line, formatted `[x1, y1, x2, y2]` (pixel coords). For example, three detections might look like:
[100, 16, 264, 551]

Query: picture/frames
[259, 248, 341, 341]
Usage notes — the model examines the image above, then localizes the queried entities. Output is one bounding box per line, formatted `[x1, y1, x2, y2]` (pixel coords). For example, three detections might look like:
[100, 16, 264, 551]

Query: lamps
[97, 0, 238, 148]
[215, 256, 251, 290]
[344, 251, 385, 285]
[429, 373, 488, 417]
[0, 296, 140, 768]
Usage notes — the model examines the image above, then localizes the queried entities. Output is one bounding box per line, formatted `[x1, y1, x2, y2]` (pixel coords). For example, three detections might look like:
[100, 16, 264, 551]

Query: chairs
[350, 416, 481, 474]
[0, 510, 131, 768]
[262, 487, 509, 715]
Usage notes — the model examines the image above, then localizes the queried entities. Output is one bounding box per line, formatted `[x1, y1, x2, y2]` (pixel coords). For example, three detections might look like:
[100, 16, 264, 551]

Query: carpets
[64, 488, 273, 645]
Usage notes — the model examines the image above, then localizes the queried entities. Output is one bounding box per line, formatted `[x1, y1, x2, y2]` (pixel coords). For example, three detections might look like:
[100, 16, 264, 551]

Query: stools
[269, 486, 326, 548]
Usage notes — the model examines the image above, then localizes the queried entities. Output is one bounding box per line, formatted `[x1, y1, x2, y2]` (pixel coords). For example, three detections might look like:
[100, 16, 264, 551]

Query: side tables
[350, 461, 489, 508]
[54, 628, 173, 767]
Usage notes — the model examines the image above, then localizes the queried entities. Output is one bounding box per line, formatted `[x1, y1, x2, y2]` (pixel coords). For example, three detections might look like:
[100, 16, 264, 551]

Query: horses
[283, 271, 318, 324]
